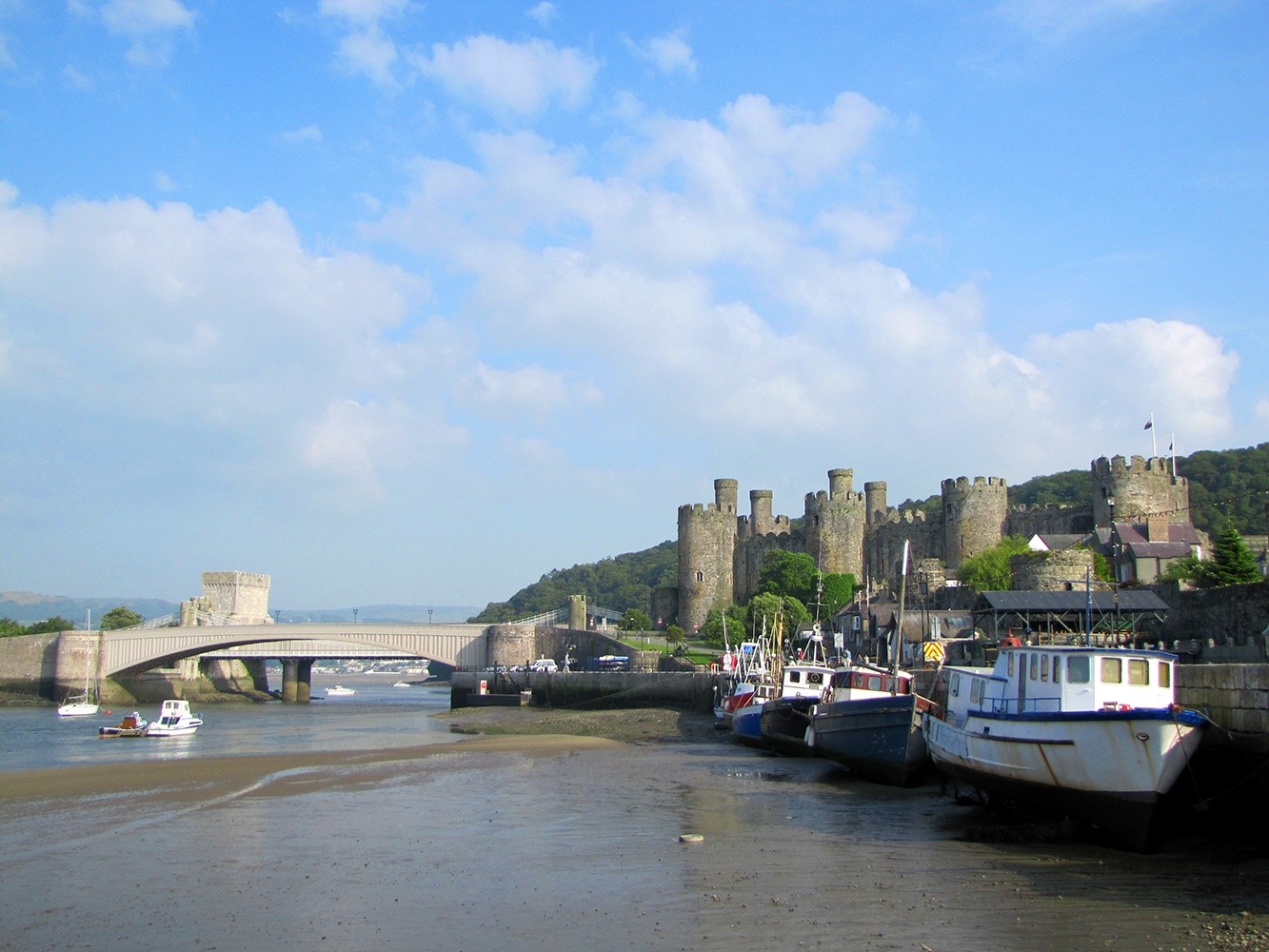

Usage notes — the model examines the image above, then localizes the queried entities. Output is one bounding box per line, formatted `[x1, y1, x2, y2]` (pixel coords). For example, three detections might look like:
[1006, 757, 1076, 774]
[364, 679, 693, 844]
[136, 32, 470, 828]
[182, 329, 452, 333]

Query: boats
[712, 536, 1213, 807]
[146, 699, 204, 736]
[324, 685, 356, 696]
[392, 680, 411, 689]
[96, 710, 148, 739]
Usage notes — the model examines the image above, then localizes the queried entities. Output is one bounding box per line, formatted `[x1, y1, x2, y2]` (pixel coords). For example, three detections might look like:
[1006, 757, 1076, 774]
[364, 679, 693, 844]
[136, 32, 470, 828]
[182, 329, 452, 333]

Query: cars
[536, 663, 558, 673]
[570, 663, 586, 672]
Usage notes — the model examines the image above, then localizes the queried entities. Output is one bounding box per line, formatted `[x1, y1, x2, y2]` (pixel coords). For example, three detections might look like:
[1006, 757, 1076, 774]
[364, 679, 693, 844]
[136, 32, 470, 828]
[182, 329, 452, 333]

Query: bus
[593, 656, 630, 672]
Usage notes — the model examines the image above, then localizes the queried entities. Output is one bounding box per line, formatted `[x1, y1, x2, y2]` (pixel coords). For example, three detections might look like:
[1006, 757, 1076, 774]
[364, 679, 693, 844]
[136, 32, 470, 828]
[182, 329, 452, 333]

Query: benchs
[1168, 640, 1202, 665]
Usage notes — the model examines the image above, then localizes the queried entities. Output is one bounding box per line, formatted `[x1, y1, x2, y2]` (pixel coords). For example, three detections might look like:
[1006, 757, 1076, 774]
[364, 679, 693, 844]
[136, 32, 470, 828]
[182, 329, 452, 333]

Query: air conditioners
[853, 616, 860, 630]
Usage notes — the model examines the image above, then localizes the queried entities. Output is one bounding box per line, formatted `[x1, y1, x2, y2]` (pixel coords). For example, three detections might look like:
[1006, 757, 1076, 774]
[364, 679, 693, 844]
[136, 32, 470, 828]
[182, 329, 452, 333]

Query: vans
[530, 659, 555, 672]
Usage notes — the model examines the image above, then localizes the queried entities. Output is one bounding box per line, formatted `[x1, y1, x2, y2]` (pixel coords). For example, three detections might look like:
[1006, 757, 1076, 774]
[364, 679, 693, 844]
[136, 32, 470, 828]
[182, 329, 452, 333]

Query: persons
[829, 648, 869, 671]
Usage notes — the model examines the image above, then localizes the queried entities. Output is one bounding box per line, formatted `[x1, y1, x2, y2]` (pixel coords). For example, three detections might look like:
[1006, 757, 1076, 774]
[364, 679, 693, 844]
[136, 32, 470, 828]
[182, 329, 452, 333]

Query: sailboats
[57, 609, 101, 716]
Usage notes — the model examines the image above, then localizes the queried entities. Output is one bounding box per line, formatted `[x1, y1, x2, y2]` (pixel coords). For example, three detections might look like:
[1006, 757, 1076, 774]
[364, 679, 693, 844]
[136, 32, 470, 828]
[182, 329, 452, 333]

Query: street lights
[428, 609, 433, 625]
[275, 610, 280, 624]
[353, 609, 358, 623]
